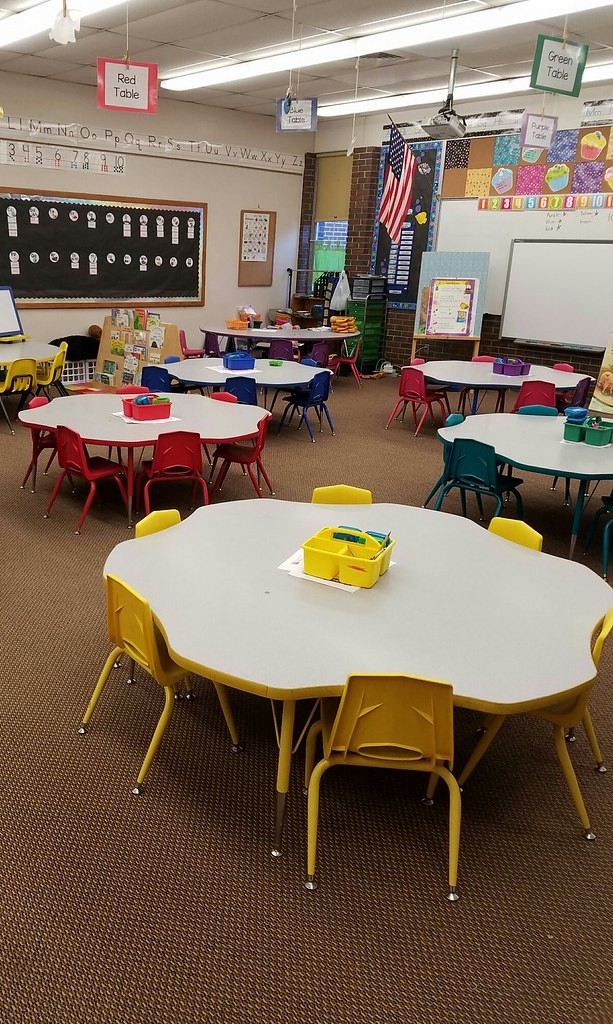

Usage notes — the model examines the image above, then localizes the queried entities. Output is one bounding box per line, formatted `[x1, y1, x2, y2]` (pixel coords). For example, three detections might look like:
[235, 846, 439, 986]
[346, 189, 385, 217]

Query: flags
[378, 122, 421, 247]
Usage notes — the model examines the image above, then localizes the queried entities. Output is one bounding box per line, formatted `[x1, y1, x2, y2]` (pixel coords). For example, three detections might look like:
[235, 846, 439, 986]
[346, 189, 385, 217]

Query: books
[93, 307, 165, 389]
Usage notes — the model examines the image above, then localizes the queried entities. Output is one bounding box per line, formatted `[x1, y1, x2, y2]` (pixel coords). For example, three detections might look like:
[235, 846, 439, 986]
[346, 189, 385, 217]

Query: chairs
[0, 330, 613, 901]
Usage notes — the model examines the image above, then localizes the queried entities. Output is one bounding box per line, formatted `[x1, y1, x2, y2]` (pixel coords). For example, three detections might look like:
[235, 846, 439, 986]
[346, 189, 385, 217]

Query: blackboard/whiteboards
[498, 239, 613, 350]
[0, 186, 208, 308]
[434, 193, 613, 315]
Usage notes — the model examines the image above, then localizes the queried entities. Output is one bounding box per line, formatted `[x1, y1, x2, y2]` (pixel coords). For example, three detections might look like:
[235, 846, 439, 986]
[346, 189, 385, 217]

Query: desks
[436, 412, 613, 560]
[199, 325, 361, 357]
[17, 393, 273, 528]
[0, 341, 61, 421]
[145, 356, 334, 413]
[102, 498, 613, 857]
[401, 361, 596, 415]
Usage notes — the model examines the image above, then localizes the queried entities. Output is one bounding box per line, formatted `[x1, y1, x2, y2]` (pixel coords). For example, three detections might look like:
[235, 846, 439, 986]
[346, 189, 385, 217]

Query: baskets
[86, 359, 98, 383]
[37, 359, 85, 384]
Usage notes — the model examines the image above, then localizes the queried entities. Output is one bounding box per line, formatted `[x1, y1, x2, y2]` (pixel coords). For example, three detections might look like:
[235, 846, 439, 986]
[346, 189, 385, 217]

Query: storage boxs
[85, 358, 97, 384]
[47, 360, 86, 386]
